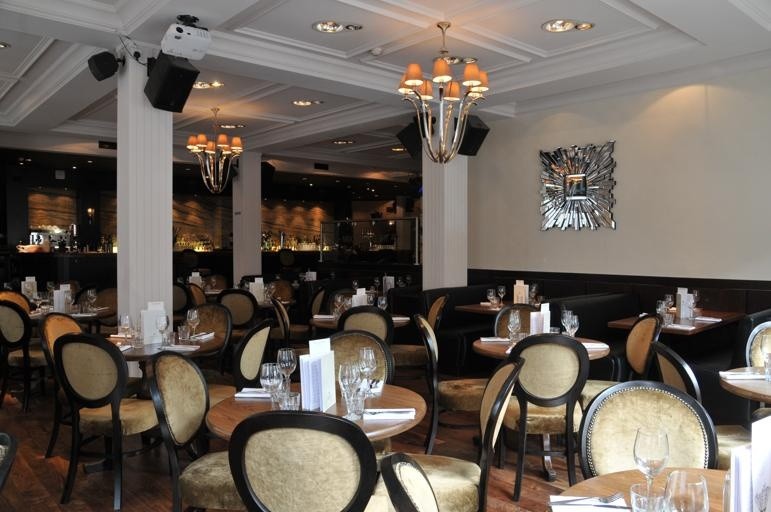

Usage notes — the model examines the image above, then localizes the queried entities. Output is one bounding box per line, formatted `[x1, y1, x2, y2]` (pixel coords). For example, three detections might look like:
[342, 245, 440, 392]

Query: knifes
[553, 501, 632, 510]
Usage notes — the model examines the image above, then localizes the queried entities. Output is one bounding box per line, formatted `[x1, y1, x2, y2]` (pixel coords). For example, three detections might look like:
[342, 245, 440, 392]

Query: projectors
[161, 24, 211, 60]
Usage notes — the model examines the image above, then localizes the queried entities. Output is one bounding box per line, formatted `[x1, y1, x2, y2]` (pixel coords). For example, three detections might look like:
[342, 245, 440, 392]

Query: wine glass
[242, 273, 298, 305]
[34, 289, 97, 316]
[334, 276, 412, 320]
[261, 348, 371, 422]
[119, 309, 200, 350]
[761, 335, 771, 380]
[656, 290, 701, 326]
[177, 274, 217, 293]
[631, 426, 709, 512]
[488, 283, 581, 346]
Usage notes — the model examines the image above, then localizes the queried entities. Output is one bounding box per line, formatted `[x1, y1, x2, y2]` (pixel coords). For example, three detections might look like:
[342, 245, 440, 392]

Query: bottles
[176, 238, 215, 252]
[18, 240, 23, 253]
[359, 226, 394, 252]
[83, 235, 113, 254]
[261, 234, 320, 252]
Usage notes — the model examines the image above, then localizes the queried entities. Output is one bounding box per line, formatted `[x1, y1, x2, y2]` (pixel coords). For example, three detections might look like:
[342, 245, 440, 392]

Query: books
[513, 279, 529, 305]
[140, 301, 167, 344]
[54, 283, 73, 313]
[249, 277, 265, 303]
[188, 271, 202, 288]
[383, 272, 396, 297]
[22, 276, 39, 300]
[529, 301, 551, 338]
[351, 288, 368, 307]
[731, 416, 771, 511]
[306, 270, 318, 281]
[676, 286, 695, 320]
[300, 337, 337, 414]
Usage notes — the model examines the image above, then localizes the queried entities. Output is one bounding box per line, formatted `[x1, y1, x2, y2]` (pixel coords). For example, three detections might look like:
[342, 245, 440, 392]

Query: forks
[192, 329, 212, 339]
[363, 409, 414, 416]
[547, 491, 625, 505]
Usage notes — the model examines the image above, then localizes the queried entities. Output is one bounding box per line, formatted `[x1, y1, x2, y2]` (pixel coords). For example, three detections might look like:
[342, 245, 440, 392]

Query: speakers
[223, 158, 237, 182]
[88, 52, 119, 82]
[262, 162, 275, 188]
[143, 50, 199, 113]
[413, 112, 434, 137]
[397, 124, 422, 157]
[452, 114, 490, 156]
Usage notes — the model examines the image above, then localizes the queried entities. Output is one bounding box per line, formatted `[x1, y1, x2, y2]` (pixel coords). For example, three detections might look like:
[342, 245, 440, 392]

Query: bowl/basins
[15, 244, 39, 253]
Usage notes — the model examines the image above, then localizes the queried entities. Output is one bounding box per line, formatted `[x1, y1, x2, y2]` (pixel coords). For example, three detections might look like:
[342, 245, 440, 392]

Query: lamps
[184, 108, 244, 195]
[396, 20, 491, 165]
